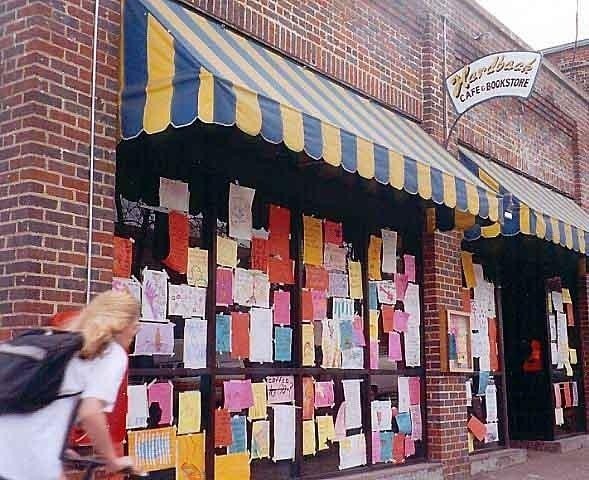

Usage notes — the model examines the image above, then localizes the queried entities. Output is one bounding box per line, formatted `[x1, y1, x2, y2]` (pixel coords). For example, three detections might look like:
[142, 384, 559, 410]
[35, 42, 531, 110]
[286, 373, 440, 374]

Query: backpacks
[0, 328, 84, 413]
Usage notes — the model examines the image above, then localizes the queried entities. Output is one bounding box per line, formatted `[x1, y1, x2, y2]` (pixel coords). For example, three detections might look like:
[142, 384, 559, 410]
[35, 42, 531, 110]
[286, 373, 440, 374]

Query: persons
[0, 288, 143, 480]
